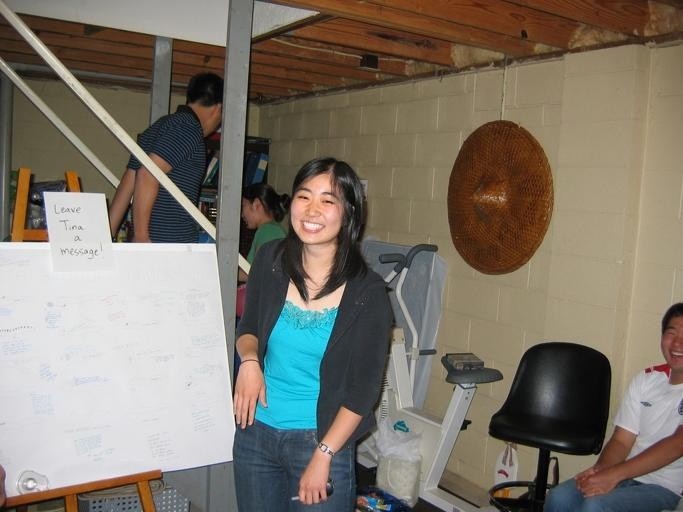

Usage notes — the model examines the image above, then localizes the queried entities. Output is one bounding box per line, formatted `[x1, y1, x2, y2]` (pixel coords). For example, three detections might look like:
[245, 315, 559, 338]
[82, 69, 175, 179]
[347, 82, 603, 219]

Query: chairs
[486, 340, 612, 511]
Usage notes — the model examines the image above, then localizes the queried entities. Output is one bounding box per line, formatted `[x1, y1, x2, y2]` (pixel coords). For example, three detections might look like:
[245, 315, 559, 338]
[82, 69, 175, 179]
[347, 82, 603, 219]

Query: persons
[544, 302, 683, 512]
[238, 183, 291, 282]
[108, 70, 223, 243]
[233, 158, 394, 512]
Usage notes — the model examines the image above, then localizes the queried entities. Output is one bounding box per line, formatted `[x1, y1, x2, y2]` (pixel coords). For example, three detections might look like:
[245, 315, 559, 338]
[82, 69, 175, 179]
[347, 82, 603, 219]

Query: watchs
[319, 442, 335, 456]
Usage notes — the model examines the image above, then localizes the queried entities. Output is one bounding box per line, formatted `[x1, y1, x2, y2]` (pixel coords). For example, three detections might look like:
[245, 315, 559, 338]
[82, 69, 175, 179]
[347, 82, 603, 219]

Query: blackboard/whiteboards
[0, 241, 236, 508]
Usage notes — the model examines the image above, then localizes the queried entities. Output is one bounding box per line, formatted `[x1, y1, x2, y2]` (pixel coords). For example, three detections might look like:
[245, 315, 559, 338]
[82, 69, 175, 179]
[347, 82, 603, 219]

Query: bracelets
[240, 360, 260, 364]
